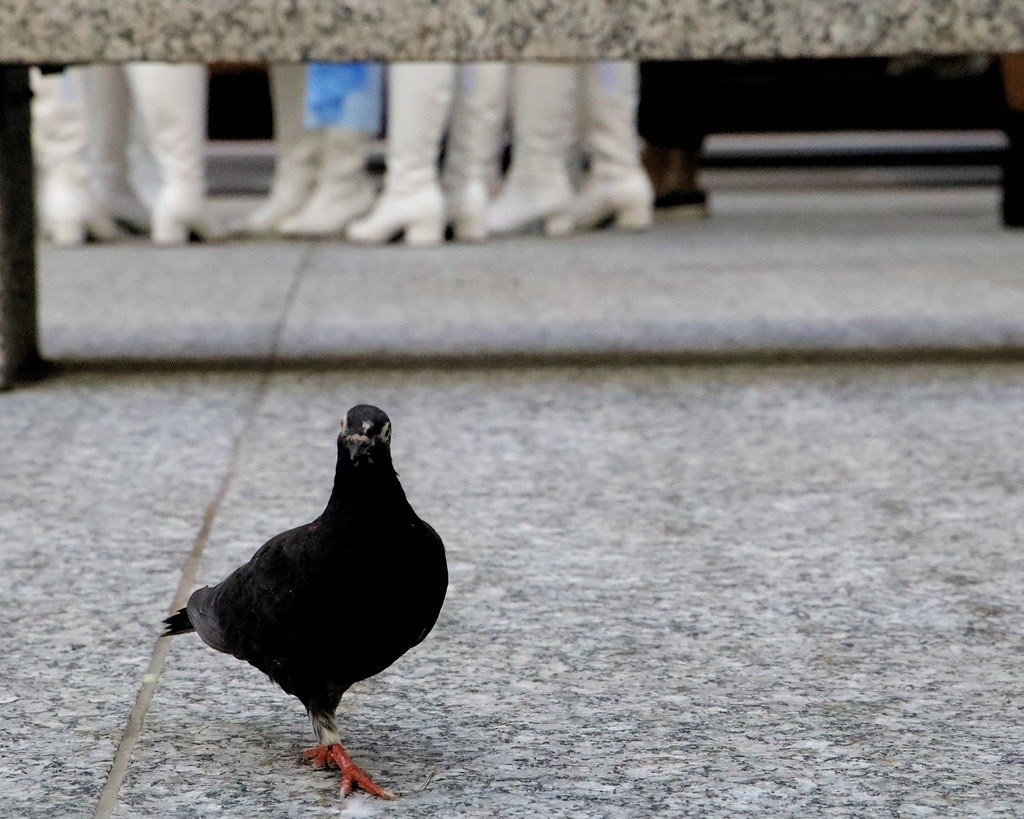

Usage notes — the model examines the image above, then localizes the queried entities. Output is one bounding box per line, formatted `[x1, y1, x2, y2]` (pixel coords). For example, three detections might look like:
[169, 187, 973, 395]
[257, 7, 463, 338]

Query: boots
[244, 61, 654, 247]
[30, 62, 131, 247]
[122, 63, 245, 247]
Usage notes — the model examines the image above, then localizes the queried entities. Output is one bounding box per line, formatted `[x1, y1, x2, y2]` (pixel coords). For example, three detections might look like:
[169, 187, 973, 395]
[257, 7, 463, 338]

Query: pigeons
[154, 404, 449, 802]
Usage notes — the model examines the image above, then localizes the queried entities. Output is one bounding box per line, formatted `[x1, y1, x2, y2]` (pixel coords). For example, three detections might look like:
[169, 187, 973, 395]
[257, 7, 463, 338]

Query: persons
[28, 58, 711, 256]
[1000, 50, 1024, 231]
